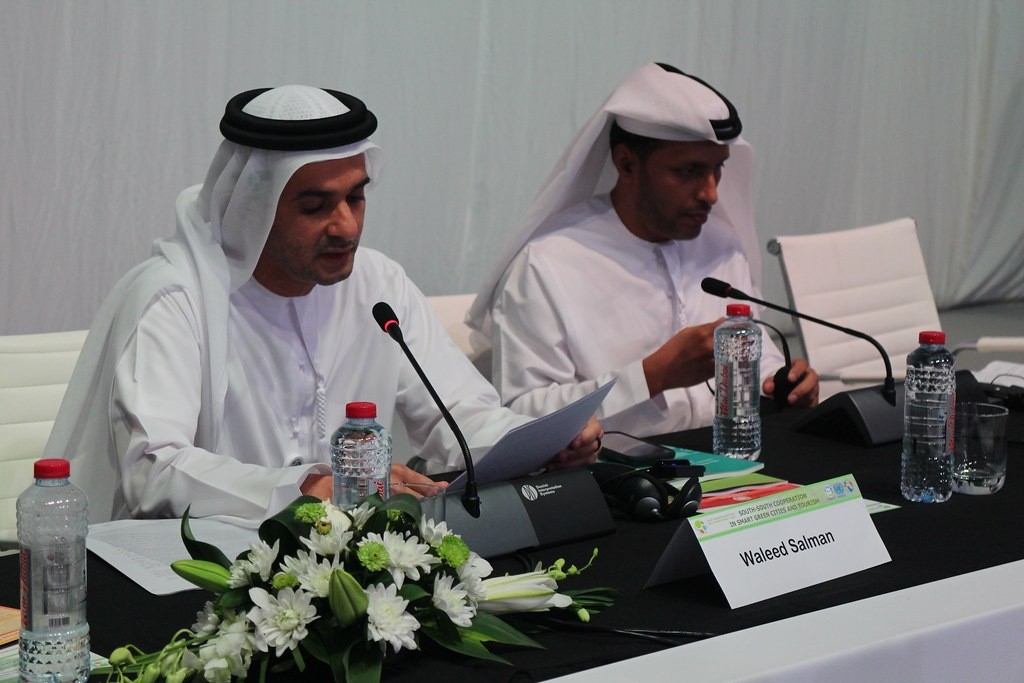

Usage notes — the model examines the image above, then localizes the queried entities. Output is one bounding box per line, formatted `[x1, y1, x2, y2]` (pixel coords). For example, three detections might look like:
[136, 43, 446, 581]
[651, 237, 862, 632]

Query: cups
[953, 403, 1008, 495]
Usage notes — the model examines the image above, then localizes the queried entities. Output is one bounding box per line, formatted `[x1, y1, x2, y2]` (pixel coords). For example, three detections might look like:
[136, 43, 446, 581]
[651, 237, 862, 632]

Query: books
[659, 445, 806, 512]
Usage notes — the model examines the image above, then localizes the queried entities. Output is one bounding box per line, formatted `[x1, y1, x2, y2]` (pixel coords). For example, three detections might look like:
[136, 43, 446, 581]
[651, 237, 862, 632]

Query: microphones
[699, 276, 992, 446]
[370, 301, 611, 563]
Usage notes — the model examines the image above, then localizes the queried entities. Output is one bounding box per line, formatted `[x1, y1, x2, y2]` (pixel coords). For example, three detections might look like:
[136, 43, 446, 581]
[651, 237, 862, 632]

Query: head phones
[704, 320, 797, 419]
[591, 462, 708, 525]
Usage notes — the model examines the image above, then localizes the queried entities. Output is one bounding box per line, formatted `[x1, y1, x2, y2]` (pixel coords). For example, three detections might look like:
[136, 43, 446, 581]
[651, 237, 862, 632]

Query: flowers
[86, 489, 619, 683]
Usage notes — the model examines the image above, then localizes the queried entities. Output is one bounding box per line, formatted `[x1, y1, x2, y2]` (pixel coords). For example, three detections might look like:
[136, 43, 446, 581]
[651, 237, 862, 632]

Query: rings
[595, 436, 601, 452]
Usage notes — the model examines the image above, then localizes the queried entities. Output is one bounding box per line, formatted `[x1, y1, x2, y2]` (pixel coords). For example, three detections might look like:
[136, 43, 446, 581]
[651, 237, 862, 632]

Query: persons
[39, 84, 603, 521]
[462, 62, 820, 453]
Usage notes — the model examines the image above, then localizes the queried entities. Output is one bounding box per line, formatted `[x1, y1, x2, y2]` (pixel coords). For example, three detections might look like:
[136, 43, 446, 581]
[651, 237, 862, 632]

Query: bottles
[901, 331, 955, 502]
[713, 304, 763, 462]
[330, 402, 392, 558]
[16, 459, 90, 683]
